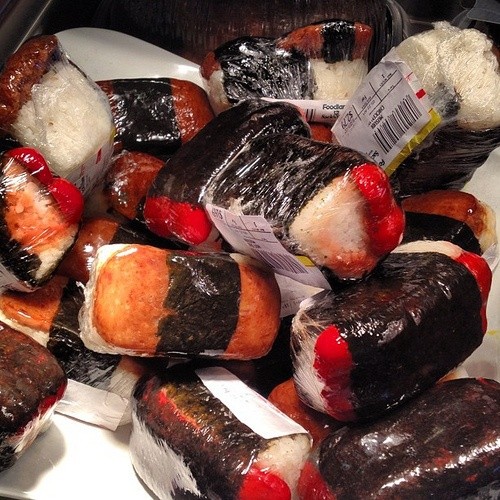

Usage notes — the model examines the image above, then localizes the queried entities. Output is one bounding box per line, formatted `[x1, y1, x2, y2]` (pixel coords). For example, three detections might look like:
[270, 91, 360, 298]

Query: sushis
[1, 0, 499, 499]
